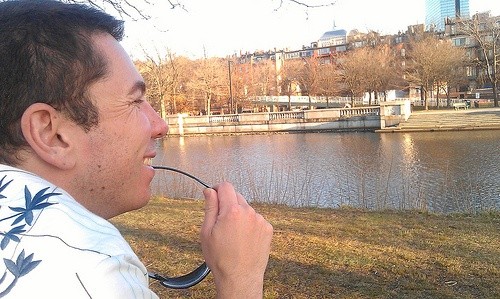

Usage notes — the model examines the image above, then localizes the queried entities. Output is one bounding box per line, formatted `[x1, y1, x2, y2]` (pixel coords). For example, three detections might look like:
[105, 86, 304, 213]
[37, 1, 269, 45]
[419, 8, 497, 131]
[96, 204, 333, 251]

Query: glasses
[148, 166, 218, 289]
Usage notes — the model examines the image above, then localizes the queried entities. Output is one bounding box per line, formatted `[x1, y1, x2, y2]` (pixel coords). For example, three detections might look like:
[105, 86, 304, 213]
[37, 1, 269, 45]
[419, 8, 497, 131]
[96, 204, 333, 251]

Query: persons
[0, 0, 273, 299]
